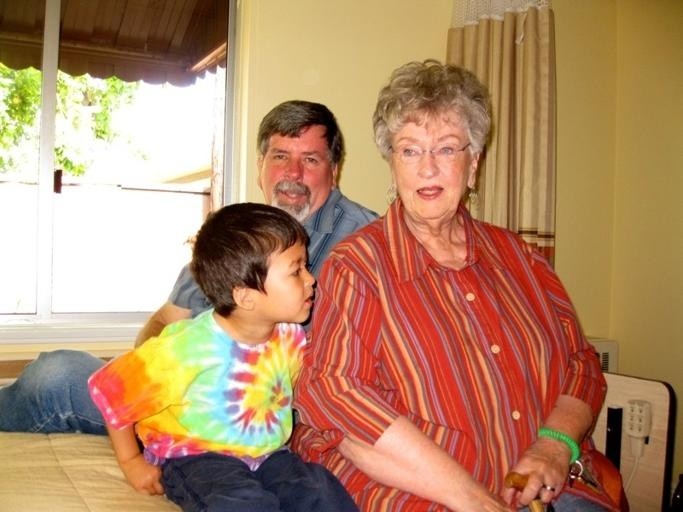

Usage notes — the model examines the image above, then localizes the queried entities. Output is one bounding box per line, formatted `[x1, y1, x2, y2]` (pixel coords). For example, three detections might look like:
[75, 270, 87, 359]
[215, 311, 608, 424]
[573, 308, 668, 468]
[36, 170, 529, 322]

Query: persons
[84, 201, 359, 512]
[0, 100, 383, 434]
[286, 57, 632, 511]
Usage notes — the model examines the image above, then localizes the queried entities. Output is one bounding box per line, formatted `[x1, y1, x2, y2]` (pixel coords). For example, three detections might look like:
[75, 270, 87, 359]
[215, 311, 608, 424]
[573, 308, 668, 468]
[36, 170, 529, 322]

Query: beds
[0, 370, 678, 509]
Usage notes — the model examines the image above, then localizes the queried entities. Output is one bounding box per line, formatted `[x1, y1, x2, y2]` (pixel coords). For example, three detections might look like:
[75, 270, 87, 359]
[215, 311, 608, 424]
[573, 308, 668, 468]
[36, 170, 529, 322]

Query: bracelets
[535, 421, 582, 465]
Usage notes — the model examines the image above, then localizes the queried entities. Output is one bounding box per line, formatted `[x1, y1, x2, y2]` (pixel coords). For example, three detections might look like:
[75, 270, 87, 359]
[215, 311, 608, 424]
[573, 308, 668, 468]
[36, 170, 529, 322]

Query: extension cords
[626, 400, 650, 438]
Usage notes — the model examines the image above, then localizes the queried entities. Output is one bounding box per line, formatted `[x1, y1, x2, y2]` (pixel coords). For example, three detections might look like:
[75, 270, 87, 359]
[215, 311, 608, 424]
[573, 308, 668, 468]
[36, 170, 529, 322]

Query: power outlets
[624, 397, 651, 438]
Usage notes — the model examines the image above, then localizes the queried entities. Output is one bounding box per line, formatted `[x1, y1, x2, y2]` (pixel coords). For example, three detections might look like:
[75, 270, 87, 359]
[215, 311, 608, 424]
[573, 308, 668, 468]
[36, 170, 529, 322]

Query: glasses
[389, 142, 470, 163]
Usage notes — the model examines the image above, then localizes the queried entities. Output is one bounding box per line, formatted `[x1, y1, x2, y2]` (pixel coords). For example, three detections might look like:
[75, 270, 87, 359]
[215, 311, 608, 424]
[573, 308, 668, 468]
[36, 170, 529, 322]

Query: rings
[543, 482, 555, 494]
